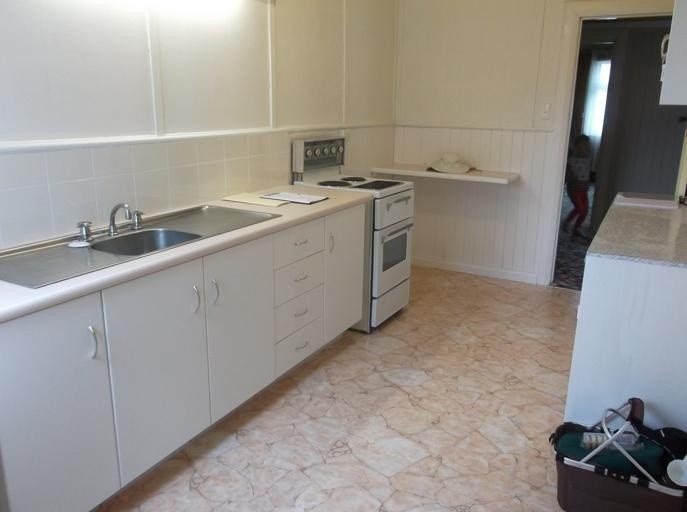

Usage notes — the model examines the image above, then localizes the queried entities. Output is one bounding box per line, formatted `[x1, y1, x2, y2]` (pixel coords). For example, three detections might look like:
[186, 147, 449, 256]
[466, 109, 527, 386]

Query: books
[259, 190, 328, 206]
[221, 192, 288, 208]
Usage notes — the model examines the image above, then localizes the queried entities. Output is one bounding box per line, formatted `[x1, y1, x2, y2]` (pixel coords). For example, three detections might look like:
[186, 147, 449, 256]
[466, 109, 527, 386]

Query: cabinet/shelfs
[325, 204, 365, 344]
[101, 234, 277, 489]
[272, 217, 325, 378]
[658, 0, 687, 105]
[563, 191, 687, 430]
[0, 291, 121, 512]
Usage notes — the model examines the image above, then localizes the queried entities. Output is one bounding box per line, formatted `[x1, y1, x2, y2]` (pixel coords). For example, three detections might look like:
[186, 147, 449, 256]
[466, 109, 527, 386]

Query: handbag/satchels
[549, 398, 687, 512]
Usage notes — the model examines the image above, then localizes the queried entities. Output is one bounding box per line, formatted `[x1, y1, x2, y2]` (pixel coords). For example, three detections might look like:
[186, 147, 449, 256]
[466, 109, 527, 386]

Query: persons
[559, 134, 591, 238]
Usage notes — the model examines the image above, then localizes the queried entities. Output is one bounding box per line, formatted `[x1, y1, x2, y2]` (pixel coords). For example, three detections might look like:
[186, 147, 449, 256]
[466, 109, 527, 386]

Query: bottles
[578, 432, 638, 451]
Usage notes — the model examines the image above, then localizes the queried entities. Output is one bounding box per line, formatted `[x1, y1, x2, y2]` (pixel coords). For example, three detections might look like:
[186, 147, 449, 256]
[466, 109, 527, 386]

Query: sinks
[90, 228, 202, 256]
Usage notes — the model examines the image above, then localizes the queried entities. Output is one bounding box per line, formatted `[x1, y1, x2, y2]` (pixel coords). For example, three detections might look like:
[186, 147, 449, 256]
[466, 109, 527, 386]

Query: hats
[429, 151, 472, 174]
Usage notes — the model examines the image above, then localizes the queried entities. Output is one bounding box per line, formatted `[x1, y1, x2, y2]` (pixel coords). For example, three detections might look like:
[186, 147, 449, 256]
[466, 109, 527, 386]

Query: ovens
[349, 182, 415, 334]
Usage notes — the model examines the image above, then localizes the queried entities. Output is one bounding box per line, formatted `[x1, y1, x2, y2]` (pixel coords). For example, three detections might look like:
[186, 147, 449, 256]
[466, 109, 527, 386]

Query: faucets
[109, 203, 132, 236]
